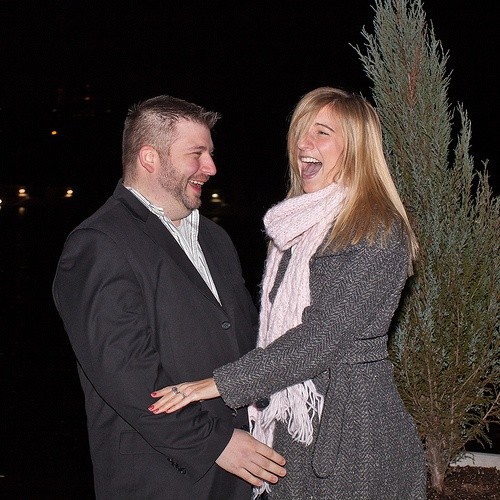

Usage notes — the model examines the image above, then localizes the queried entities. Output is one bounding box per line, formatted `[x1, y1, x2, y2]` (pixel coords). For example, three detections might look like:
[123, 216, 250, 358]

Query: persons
[147, 88, 428, 500]
[51, 97, 286, 500]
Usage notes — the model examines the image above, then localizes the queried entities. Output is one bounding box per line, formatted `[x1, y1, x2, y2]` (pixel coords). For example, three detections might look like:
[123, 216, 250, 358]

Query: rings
[172, 387, 178, 393]
[179, 392, 185, 397]
